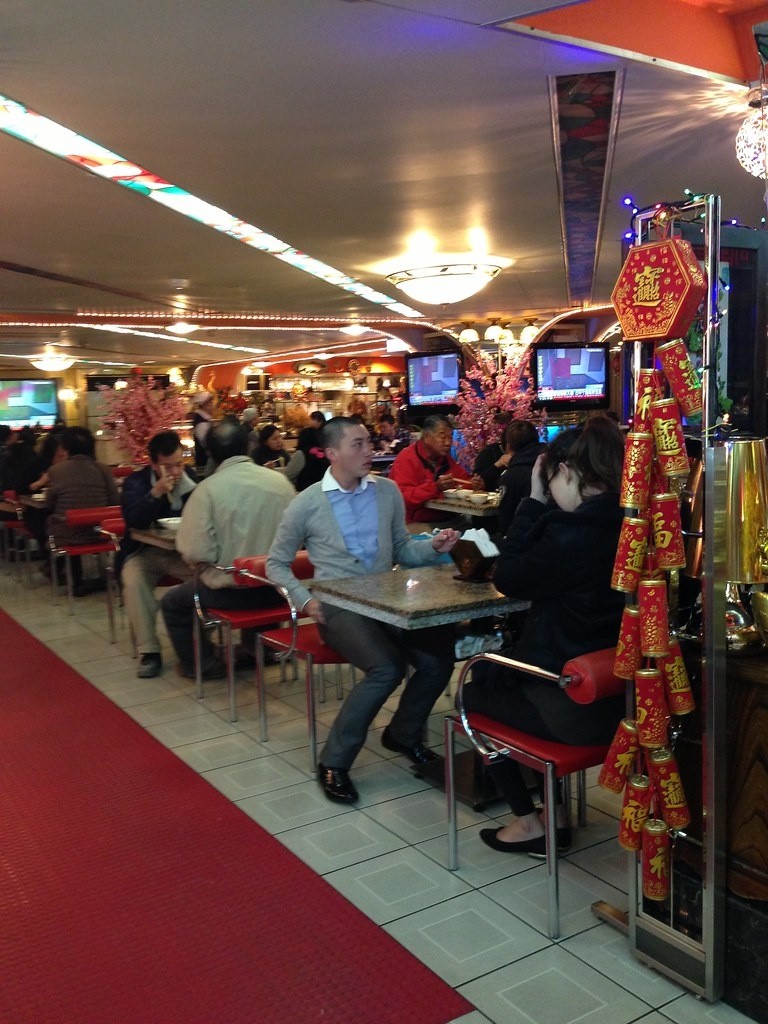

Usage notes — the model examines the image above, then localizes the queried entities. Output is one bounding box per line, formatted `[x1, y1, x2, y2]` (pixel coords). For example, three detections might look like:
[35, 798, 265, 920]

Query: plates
[274, 467, 286, 471]
[156, 517, 182, 530]
[486, 493, 500, 502]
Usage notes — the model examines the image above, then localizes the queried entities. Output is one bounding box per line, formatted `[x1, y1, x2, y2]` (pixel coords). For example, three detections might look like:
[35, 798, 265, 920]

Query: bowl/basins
[470, 494, 487, 505]
[456, 489, 473, 500]
[442, 489, 458, 499]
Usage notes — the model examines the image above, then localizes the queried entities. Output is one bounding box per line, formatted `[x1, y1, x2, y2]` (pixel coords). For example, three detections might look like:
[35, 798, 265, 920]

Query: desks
[129, 528, 294, 670]
[311, 562, 563, 813]
[16, 492, 50, 509]
[427, 494, 503, 524]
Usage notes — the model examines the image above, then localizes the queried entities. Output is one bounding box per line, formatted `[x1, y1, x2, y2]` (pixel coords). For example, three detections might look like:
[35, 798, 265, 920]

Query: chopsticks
[159, 465, 174, 503]
[438, 475, 472, 485]
[264, 459, 281, 465]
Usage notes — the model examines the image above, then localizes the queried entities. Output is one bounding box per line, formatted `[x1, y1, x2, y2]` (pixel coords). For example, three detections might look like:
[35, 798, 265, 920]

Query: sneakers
[137, 654, 161, 678]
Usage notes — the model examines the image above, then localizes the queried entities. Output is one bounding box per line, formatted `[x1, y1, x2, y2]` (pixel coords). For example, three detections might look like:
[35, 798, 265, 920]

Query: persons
[265, 416, 461, 804]
[389, 416, 484, 534]
[472, 428, 511, 490]
[351, 413, 411, 455]
[251, 425, 290, 469]
[375, 378, 392, 401]
[0, 420, 120, 597]
[310, 412, 326, 427]
[347, 395, 367, 415]
[160, 420, 297, 680]
[193, 391, 213, 465]
[454, 416, 626, 858]
[113, 430, 204, 678]
[463, 418, 548, 638]
[222, 408, 259, 454]
[284, 427, 331, 493]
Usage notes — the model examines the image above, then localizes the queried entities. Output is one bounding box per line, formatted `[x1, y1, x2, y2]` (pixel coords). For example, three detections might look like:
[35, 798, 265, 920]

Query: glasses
[541, 460, 571, 495]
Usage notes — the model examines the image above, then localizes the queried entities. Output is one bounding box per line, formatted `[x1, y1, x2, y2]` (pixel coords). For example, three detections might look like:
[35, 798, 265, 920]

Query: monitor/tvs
[0, 377, 59, 432]
[529, 342, 611, 412]
[405, 348, 465, 413]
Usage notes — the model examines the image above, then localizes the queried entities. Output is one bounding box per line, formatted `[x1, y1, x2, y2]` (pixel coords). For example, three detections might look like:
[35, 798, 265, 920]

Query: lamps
[338, 323, 372, 335]
[385, 263, 504, 304]
[446, 317, 540, 344]
[30, 353, 77, 371]
[164, 321, 200, 335]
[735, 106, 768, 180]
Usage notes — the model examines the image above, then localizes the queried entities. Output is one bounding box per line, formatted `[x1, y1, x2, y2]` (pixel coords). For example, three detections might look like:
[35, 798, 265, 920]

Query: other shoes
[535, 807, 570, 851]
[71, 580, 87, 597]
[174, 659, 225, 678]
[39, 566, 68, 586]
[480, 826, 558, 859]
[233, 640, 267, 658]
[28, 538, 40, 551]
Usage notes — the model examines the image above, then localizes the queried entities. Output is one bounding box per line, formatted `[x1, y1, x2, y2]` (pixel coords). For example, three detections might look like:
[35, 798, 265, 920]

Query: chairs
[0, 467, 625, 940]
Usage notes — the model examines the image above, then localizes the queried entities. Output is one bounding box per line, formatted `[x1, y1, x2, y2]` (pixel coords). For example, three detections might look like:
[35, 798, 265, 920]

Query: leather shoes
[317, 763, 358, 804]
[381, 725, 444, 771]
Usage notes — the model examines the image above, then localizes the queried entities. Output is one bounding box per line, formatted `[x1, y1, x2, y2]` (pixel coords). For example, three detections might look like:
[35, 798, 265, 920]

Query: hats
[193, 391, 212, 407]
